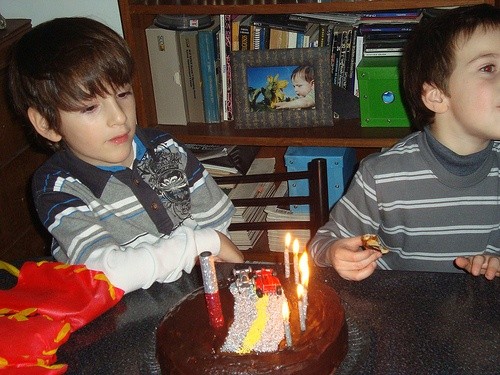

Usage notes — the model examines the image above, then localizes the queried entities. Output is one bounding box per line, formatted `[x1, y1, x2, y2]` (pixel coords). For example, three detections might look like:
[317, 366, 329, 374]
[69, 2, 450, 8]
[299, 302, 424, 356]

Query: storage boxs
[357, 58, 412, 128]
[283, 146, 358, 213]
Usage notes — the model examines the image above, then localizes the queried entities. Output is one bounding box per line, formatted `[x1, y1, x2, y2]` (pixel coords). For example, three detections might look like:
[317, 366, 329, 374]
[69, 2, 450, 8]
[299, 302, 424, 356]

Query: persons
[276, 66, 315, 109]
[306, 4, 499, 281]
[7, 18, 245, 295]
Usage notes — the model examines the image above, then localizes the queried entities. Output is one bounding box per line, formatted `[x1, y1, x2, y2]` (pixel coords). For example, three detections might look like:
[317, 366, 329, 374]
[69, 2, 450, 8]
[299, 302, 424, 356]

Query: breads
[361, 234, 389, 253]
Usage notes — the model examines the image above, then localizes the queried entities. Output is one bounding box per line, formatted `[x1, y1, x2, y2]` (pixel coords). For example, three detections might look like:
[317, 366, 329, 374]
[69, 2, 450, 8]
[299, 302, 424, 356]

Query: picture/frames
[230, 46, 335, 130]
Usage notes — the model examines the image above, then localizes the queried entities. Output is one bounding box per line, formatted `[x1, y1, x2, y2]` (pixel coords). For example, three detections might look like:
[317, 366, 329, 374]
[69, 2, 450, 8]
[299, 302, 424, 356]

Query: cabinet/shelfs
[118, 0, 500, 149]
[0, 20, 51, 279]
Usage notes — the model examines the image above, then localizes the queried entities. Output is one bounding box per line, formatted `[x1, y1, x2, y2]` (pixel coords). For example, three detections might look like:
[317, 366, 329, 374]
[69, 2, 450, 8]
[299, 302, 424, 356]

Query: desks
[56, 261, 500, 375]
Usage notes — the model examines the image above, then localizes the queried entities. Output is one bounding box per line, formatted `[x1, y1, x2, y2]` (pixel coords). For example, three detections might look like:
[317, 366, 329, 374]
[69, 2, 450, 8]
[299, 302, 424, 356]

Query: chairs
[212, 157, 329, 265]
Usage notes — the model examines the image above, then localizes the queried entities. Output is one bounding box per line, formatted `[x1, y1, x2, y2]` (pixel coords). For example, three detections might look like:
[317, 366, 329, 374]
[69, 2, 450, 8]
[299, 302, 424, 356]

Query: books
[145, 9, 423, 126]
[186, 143, 311, 252]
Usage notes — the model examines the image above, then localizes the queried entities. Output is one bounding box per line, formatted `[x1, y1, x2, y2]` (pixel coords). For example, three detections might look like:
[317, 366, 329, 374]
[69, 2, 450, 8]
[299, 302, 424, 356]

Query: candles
[282, 232, 309, 348]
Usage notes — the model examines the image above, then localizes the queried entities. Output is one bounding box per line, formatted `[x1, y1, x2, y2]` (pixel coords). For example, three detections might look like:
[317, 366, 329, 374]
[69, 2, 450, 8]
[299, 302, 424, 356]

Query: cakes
[156, 266, 350, 375]
